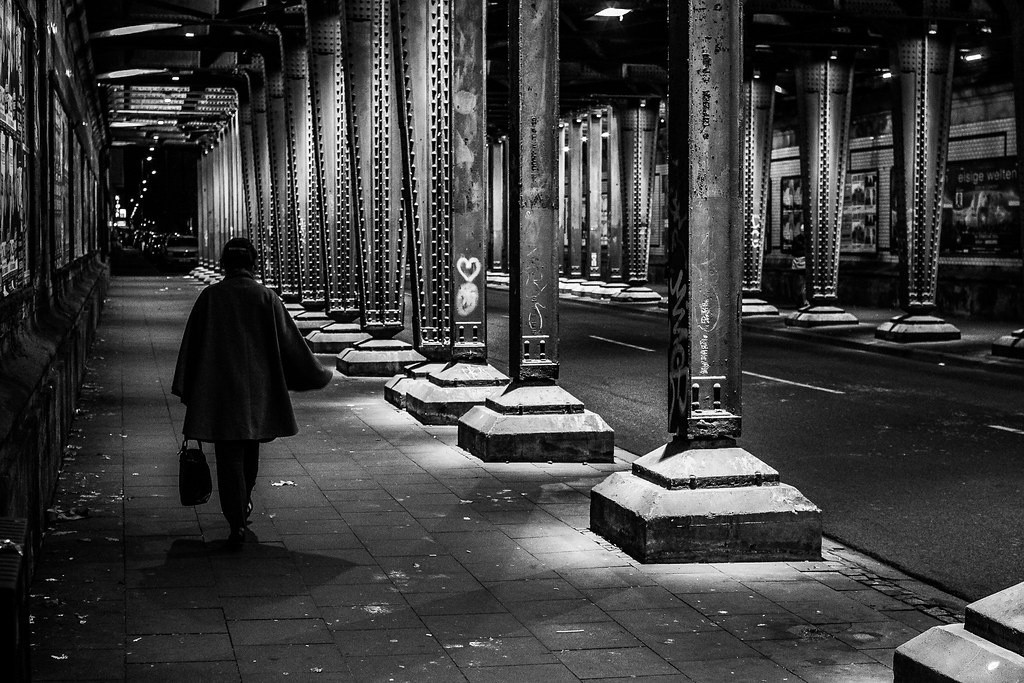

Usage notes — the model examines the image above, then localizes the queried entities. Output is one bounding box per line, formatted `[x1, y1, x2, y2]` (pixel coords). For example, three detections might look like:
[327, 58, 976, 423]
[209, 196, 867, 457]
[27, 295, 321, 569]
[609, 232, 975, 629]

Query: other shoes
[229, 500, 254, 548]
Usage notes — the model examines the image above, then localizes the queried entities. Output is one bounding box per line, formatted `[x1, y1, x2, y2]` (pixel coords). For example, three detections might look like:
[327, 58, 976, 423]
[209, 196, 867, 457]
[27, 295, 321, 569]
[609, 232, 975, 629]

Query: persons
[852, 188, 865, 220]
[790, 224, 810, 311]
[169, 237, 333, 553]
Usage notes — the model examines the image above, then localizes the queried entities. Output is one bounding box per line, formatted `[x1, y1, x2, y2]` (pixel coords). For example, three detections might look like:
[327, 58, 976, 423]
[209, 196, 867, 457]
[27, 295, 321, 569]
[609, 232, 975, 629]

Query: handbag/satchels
[180, 439, 212, 506]
